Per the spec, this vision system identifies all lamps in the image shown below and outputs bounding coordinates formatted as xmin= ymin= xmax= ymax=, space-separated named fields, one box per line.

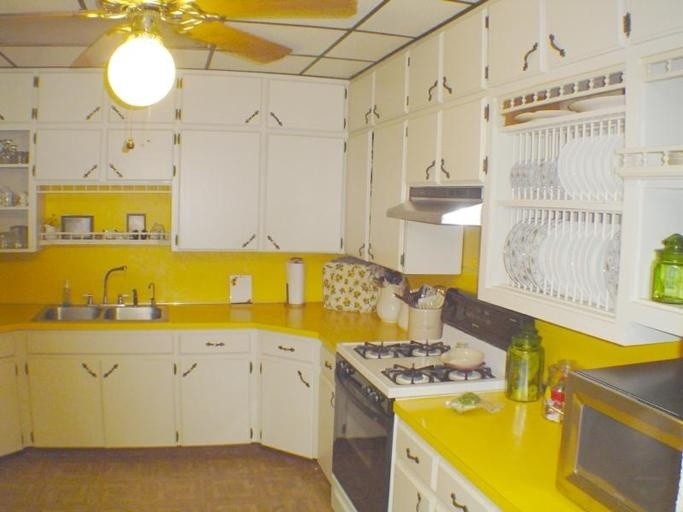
xmin=107 ymin=19 xmax=176 ymax=108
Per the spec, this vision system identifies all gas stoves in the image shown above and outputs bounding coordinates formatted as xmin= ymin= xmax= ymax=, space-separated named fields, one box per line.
xmin=336 ymin=323 xmax=506 ymax=416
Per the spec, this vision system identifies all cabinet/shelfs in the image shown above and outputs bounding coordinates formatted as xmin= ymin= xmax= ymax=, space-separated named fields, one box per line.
xmin=487 ymin=1 xmax=625 ymax=87
xmin=408 ymin=9 xmax=486 ymax=192
xmin=178 ymin=330 xmax=258 ymax=447
xmin=259 ymin=334 xmax=318 ymax=461
xmin=38 ymin=73 xmax=175 ymax=187
xmin=176 ymin=69 xmax=345 ymax=254
xmin=347 ymin=53 xmax=408 ymax=273
xmin=1 ymin=333 xmax=23 ymax=459
xmin=26 ymin=329 xmax=178 ymax=448
xmin=1 ymin=69 xmax=38 ymax=252
xmin=317 ymin=347 xmax=338 ymax=483
xmin=625 ymin=1 xmax=683 ymax=335
xmin=388 ymin=420 xmax=498 ymax=512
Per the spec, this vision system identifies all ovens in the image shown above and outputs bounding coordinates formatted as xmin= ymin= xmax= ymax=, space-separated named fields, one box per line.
xmin=332 ymin=372 xmax=395 ymax=511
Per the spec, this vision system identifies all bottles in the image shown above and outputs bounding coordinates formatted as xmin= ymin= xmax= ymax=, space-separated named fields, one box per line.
xmin=506 ymin=328 xmax=545 ymax=402
xmin=650 ymin=233 xmax=683 ymax=304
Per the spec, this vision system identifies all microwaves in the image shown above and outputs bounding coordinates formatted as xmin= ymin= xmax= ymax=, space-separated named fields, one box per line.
xmin=556 ymin=357 xmax=683 ymax=512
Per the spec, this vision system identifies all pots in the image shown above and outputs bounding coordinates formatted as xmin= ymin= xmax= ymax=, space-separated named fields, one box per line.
xmin=439 ymin=342 xmax=485 ymax=370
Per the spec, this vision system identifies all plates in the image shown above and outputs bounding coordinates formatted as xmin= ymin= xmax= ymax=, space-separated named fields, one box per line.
xmin=514 ymin=110 xmax=575 ymax=120
xmin=502 ymin=134 xmax=624 ymax=302
xmin=568 ymin=95 xmax=625 ymax=112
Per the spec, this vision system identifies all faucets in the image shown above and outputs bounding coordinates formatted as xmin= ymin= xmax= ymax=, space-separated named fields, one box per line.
xmin=102 ymin=264 xmax=127 ymax=304
xmin=148 ymin=282 xmax=156 ymax=306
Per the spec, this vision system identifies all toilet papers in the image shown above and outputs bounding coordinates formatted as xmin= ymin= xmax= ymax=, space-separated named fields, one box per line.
xmin=286 ymin=258 xmax=305 ymax=309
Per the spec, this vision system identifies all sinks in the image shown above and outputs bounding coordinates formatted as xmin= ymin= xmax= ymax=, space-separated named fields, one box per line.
xmin=104 ymin=306 xmax=162 ymax=321
xmin=35 ymin=303 xmax=102 ymax=321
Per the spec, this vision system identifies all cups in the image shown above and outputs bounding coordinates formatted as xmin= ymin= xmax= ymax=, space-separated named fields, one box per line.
xmin=408 ymin=307 xmax=443 ymax=340
xmin=376 ymin=287 xmax=402 ymax=322
xmin=397 ymin=301 xmax=409 ymax=331
xmin=542 ymin=361 xmax=581 ymax=422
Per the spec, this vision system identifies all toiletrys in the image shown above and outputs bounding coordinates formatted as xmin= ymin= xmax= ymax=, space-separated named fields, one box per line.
xmin=62 ymin=280 xmax=72 ymax=305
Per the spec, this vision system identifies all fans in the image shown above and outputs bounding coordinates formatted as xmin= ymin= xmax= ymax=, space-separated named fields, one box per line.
xmin=0 ymin=0 xmax=357 ymax=69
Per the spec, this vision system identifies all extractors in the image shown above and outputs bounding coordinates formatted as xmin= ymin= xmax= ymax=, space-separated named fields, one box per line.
xmin=386 ymin=185 xmax=483 ymax=226
